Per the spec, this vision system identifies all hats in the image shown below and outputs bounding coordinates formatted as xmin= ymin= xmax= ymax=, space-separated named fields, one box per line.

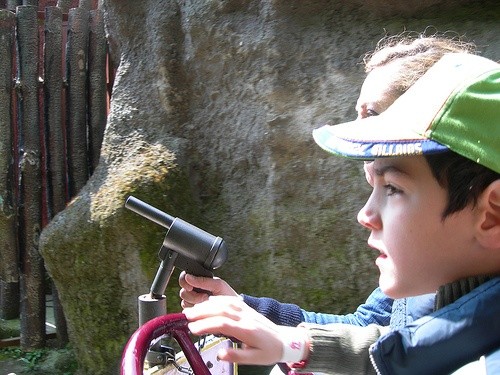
xmin=313 ymin=53 xmax=500 ymax=174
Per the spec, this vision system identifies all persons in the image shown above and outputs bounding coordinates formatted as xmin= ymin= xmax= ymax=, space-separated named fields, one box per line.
xmin=183 ymin=53 xmax=500 ymax=375
xmin=179 ymin=36 xmax=472 ymax=326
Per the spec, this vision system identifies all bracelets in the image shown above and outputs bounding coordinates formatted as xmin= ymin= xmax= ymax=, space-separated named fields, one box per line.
xmin=281 ymin=324 xmax=306 ymax=362
